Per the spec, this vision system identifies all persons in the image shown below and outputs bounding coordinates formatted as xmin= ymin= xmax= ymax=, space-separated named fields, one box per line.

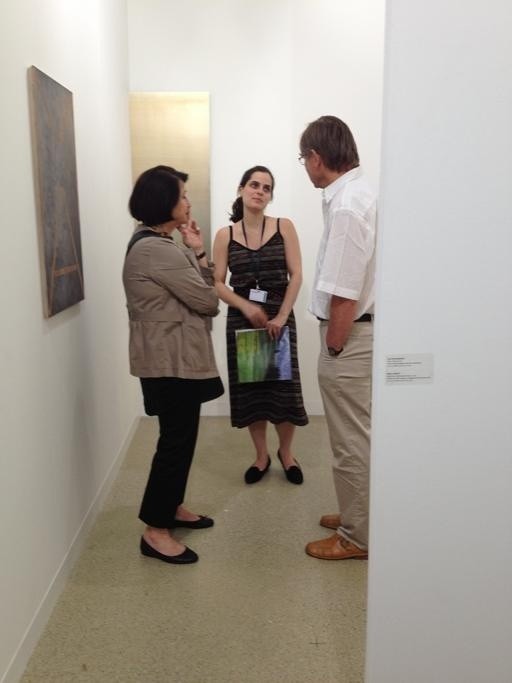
xmin=213 ymin=166 xmax=309 ymax=484
xmin=298 ymin=115 xmax=376 ymax=562
xmin=121 ymin=165 xmax=218 ymax=563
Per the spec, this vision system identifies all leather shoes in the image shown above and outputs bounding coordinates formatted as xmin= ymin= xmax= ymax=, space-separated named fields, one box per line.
xmin=245 ymin=454 xmax=271 ymax=484
xmin=305 ymin=532 xmax=368 ymax=560
xmin=140 ymin=530 xmax=199 ymax=566
xmin=277 ymin=448 xmax=303 ymax=484
xmin=319 ymin=512 xmax=343 ymax=530
xmin=172 ymin=512 xmax=214 ymax=528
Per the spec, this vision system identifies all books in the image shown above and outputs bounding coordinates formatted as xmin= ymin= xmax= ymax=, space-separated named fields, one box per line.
xmin=235 ymin=325 xmax=293 ymax=384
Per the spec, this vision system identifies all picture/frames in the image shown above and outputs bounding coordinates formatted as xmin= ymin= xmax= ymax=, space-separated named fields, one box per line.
xmin=27 ymin=64 xmax=84 ymax=320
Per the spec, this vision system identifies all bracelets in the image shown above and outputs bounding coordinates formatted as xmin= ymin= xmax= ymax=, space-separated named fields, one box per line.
xmin=197 ymin=251 xmax=206 ymax=259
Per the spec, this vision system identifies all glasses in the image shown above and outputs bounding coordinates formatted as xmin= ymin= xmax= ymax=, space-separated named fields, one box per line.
xmin=298 ymin=156 xmax=306 ymax=164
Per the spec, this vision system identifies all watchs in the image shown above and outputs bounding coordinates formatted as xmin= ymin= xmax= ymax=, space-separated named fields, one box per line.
xmin=326 ymin=347 xmax=343 ymax=356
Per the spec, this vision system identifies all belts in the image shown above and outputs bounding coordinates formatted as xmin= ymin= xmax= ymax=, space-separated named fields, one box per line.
xmin=314 ymin=314 xmax=372 ymax=323
